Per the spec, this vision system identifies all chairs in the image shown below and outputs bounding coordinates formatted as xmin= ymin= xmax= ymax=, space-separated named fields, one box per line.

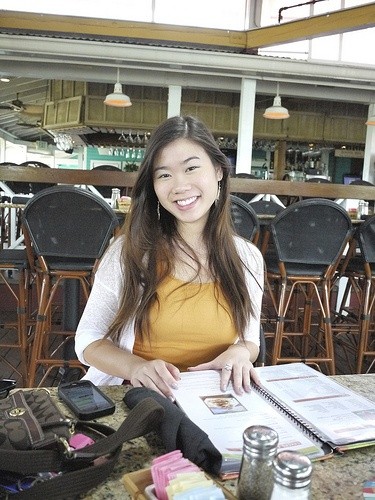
xmin=0 ymin=160 xmax=375 ymax=390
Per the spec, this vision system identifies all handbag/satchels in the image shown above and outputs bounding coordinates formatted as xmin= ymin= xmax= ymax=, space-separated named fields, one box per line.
xmin=0 ymin=388 xmax=164 ymax=500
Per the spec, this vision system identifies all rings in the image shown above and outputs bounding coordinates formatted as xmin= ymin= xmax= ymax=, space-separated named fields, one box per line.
xmin=223 ymin=364 xmax=233 ymax=371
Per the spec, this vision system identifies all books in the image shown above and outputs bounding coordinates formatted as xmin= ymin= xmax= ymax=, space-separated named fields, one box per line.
xmin=159 ymin=361 xmax=375 ymax=481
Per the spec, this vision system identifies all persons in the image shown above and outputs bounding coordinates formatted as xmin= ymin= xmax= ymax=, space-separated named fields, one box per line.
xmin=73 ymin=116 xmax=266 ymax=403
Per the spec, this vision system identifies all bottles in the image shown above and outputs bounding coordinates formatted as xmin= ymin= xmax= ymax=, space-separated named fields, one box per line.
xmin=111 ymin=188 xmax=120 ymax=209
xmin=358 ymin=202 xmax=369 ymax=220
xmin=349 ymin=208 xmax=358 ymax=220
xmin=236 ymin=426 xmax=279 ymax=500
xmin=270 ymin=450 xmax=313 ymax=500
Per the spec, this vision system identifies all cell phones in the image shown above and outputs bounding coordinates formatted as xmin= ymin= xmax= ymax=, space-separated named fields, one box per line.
xmin=59 ymin=380 xmax=117 ymax=421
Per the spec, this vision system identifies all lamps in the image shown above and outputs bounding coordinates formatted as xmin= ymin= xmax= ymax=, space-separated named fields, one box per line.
xmin=263 ymin=81 xmax=291 ymax=119
xmin=365 ymin=106 xmax=375 ymax=126
xmin=11 ymin=94 xmax=22 ymax=109
xmin=103 ymin=68 xmax=133 ymax=108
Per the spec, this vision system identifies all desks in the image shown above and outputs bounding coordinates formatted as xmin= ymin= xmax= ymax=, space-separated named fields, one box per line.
xmin=9 ymin=373 xmax=375 ymax=500
